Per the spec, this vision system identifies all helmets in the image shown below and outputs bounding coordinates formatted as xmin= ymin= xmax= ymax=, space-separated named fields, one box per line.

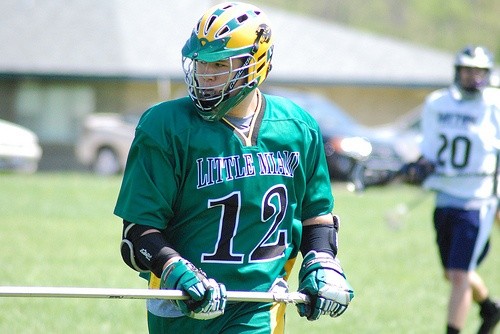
xmin=181 ymin=2 xmax=274 ymax=121
xmin=453 ymin=46 xmax=493 ymax=101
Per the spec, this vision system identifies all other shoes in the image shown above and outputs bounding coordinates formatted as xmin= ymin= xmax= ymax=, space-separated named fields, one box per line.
xmin=478 ymin=303 xmax=500 ymax=334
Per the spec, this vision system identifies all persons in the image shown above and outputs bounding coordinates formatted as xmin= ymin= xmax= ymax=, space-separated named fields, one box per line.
xmin=113 ymin=1 xmax=355 ymax=334
xmin=402 ymin=42 xmax=500 ymax=334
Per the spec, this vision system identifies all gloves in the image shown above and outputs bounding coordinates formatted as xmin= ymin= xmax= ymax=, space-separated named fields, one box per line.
xmin=159 ymin=259 xmax=228 ymax=320
xmin=295 ymin=250 xmax=355 ymax=321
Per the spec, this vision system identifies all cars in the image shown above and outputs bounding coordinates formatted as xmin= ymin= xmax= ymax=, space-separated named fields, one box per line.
xmin=260 ymin=87 xmax=397 ymax=181
xmin=74 ymin=111 xmax=147 ymax=176
xmin=379 ymin=106 xmax=432 ymax=185
xmin=1 ymin=120 xmax=43 ymax=173
